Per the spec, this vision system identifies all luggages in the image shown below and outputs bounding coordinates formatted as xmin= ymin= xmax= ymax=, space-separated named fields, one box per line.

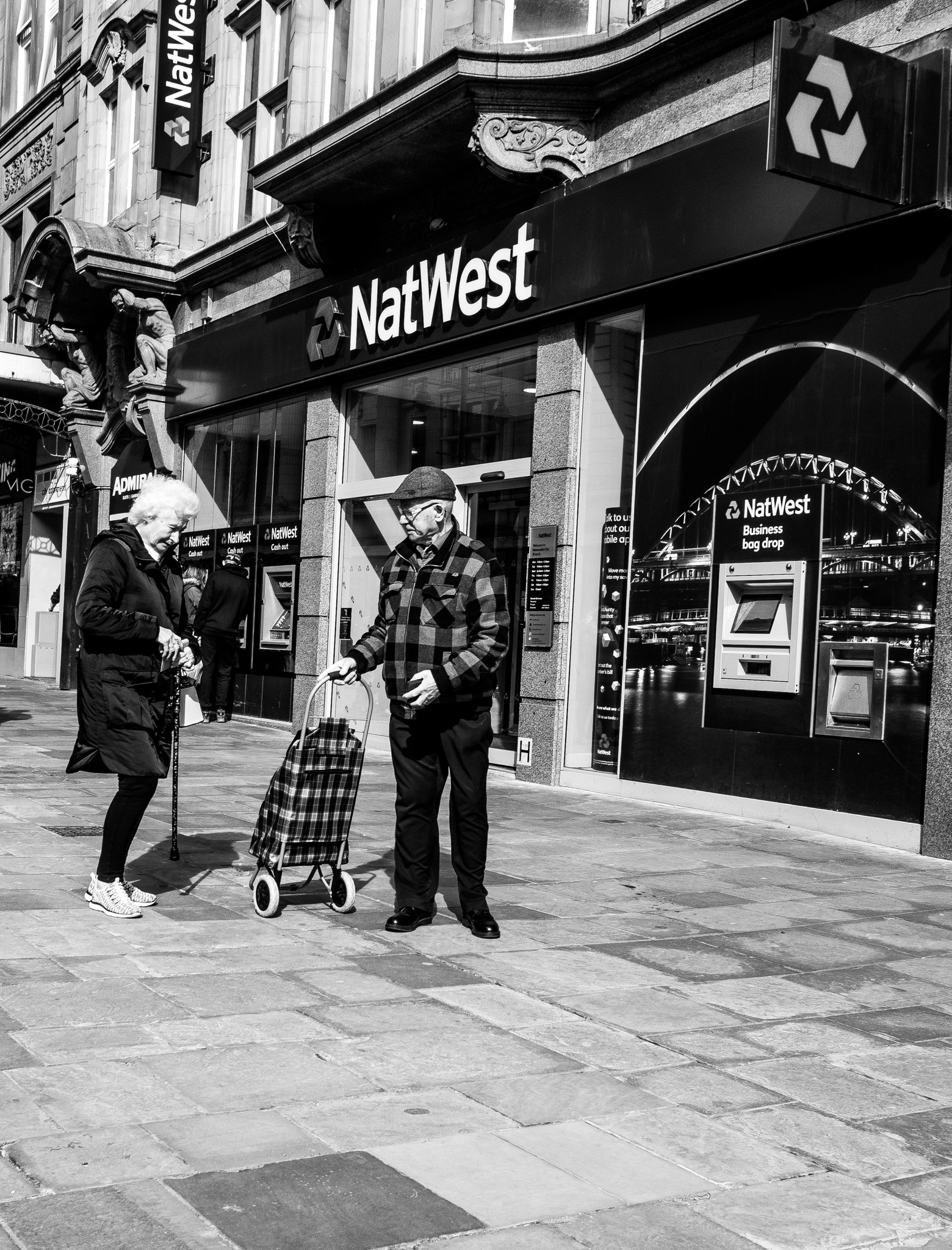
xmin=247 ymin=671 xmax=374 ymax=918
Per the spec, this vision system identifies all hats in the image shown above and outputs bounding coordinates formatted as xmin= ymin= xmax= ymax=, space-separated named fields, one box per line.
xmin=224 ymin=554 xmax=241 ymax=566
xmin=389 ymin=467 xmax=457 ymax=501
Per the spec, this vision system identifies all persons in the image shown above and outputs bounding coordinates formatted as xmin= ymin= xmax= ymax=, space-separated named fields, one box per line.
xmin=65 ymin=476 xmax=204 ymax=918
xmin=317 ymin=468 xmax=509 ymax=938
xmin=37 ymin=323 xmax=107 ymax=412
xmin=182 ymin=566 xmax=207 ymax=624
xmin=111 ymin=289 xmax=176 ymax=384
xmin=193 ymin=553 xmax=252 ymax=723
xmin=49 ymin=584 xmax=60 ymax=611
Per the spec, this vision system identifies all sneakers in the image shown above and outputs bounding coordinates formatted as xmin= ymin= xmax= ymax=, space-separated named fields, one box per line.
xmin=84 ymin=872 xmax=157 ymax=907
xmin=88 ymin=875 xmax=142 ymax=918
xmin=217 ymin=710 xmax=227 ymax=722
xmin=201 ymin=710 xmax=210 ymax=723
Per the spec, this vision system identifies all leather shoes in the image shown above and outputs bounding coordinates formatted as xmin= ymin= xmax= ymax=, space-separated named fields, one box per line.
xmin=384 ymin=905 xmax=434 ymax=930
xmin=463 ymin=907 xmax=500 ymax=938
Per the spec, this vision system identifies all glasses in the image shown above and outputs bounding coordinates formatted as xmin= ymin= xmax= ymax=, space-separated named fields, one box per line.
xmin=397 ymin=503 xmax=447 ymax=518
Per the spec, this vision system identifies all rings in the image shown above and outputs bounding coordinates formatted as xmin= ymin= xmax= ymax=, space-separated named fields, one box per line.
xmin=173 ymin=641 xmax=178 ymax=644
xmin=185 ymin=656 xmax=189 ymax=662
